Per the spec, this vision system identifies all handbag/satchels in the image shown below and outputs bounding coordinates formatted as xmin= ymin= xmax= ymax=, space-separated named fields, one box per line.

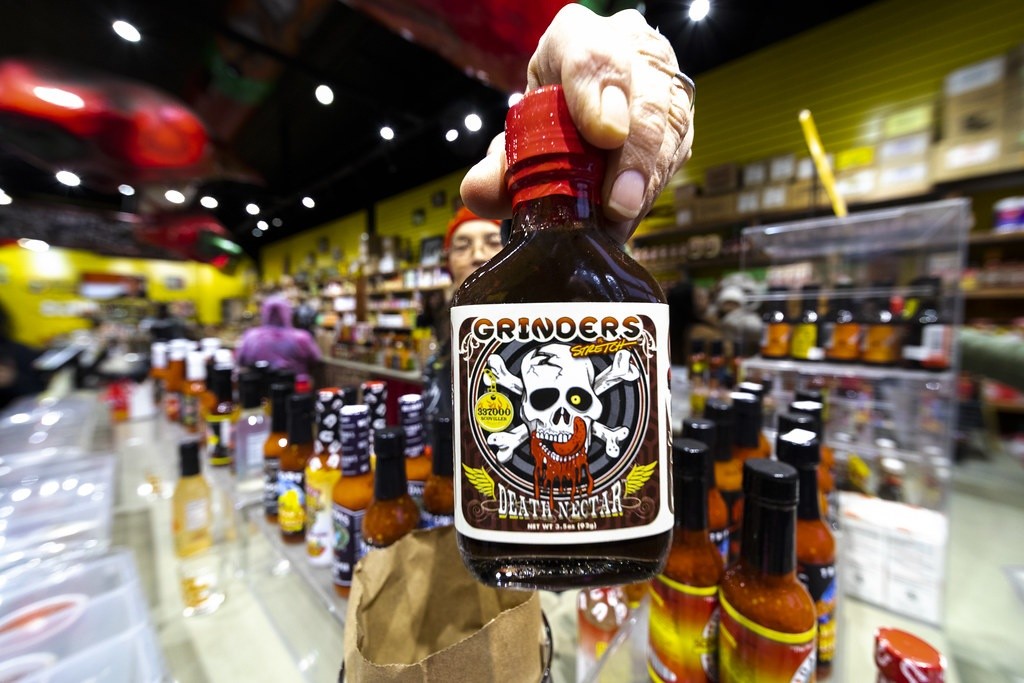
xmin=336 ymin=524 xmax=554 ymax=683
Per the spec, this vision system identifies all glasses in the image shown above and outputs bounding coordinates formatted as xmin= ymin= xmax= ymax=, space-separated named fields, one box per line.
xmin=443 ymin=233 xmax=502 ymax=254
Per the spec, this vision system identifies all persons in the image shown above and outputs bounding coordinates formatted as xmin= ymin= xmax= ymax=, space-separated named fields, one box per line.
xmin=413 ymin=0 xmax=697 ymax=287
xmin=236 ymin=296 xmax=323 ymax=378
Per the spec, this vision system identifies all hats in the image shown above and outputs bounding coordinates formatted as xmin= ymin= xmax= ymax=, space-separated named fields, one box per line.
xmin=444 ymin=206 xmax=503 ymax=251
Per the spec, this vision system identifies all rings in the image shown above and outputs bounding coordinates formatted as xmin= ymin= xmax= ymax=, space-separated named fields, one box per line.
xmin=675 ymin=71 xmax=695 ymax=111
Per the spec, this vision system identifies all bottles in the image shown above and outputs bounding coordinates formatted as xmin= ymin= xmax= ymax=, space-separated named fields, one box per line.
xmin=776 ymin=429 xmax=836 ymax=681
xmin=689 ymin=351 xmax=707 ymax=414
xmin=709 ymin=355 xmax=727 ymax=399
xmin=794 ymin=388 xmax=835 ymax=472
xmin=576 ymin=586 xmax=633 ymax=683
xmin=778 ymin=414 xmax=830 ymax=521
xmin=737 ymin=381 xmax=772 ymax=459
xmin=742 ymin=273 xmax=954 ymax=370
xmin=705 ymin=402 xmax=743 ymax=536
xmin=875 ymin=628 xmax=944 ymax=683
xmin=730 ymin=393 xmax=766 ymax=465
xmin=149 ymin=336 xmax=452 ymax=618
xmin=720 ymin=459 xmax=817 ymax=683
xmin=645 ymin=438 xmax=726 ymax=683
xmin=730 ymin=357 xmax=746 ymax=392
xmin=790 ymin=401 xmax=832 ymax=495
xmin=836 ymin=429 xmax=952 ymax=512
xmin=682 ymin=418 xmax=728 ymax=569
xmin=451 ymin=86 xmax=676 ymax=590
